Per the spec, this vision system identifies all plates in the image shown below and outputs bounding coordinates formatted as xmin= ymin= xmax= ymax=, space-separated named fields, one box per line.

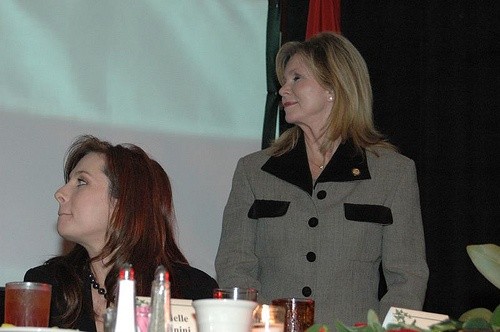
xmin=0 ymin=324 xmax=86 ymax=332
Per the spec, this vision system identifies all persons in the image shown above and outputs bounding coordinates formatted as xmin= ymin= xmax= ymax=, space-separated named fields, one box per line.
xmin=23 ymin=135 xmax=220 ymax=332
xmin=214 ymin=30 xmax=429 ymax=332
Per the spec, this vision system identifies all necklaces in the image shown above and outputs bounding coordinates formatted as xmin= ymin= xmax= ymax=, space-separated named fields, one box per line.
xmin=318 ymin=161 xmax=325 ymax=171
xmin=88 ymin=271 xmax=108 ymax=301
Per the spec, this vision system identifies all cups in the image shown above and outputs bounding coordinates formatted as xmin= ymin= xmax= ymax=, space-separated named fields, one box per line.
xmin=273 ymin=298 xmax=315 ymax=332
xmin=211 ymin=288 xmax=258 ymax=303
xmin=191 ymin=298 xmax=259 ymax=332
xmin=248 ymin=302 xmax=286 ymax=332
xmin=4 ymin=282 xmax=52 ymax=328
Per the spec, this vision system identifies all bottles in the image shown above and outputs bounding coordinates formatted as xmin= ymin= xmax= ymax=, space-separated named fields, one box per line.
xmin=109 ymin=262 xmax=138 ymax=332
xmin=149 ymin=264 xmax=172 ymax=332
